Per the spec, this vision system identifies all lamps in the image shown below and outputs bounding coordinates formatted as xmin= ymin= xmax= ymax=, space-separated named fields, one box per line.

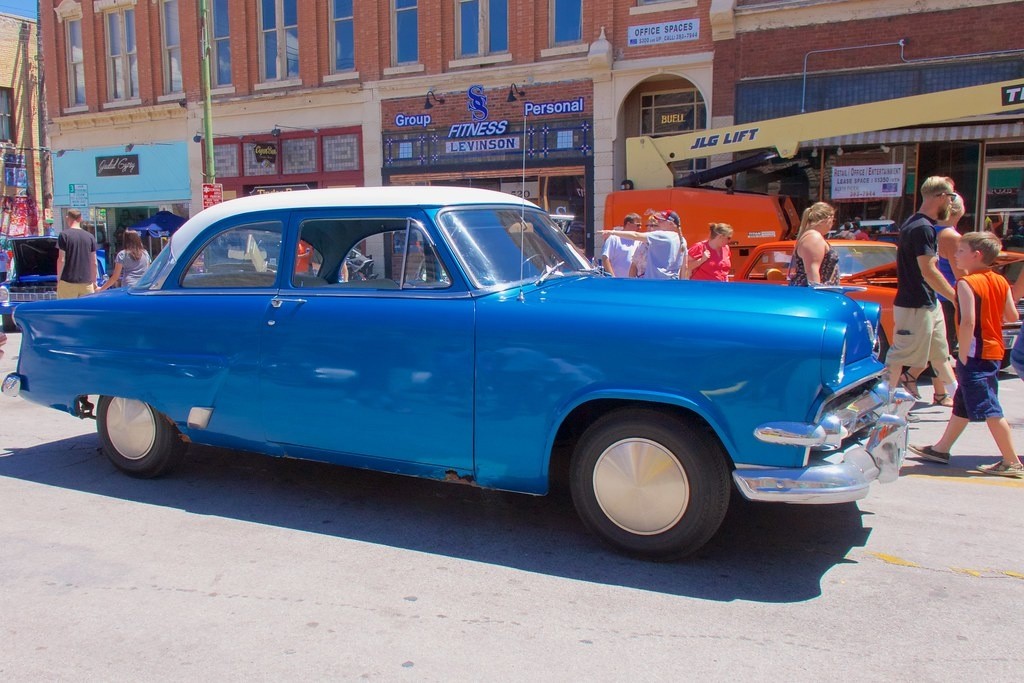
xmin=507 ymin=83 xmax=525 ymax=103
xmin=270 ymin=124 xmax=319 ymax=137
xmin=125 ymin=143 xmax=171 ymax=153
xmin=811 ymin=147 xmax=818 ymax=157
xmin=193 ymin=132 xmax=243 ymax=143
xmin=55 ymin=150 xmax=83 ymax=158
xmin=880 ymin=144 xmax=890 ymax=153
xmin=837 ymin=146 xmax=844 ymax=156
xmin=424 ymin=91 xmax=445 ymax=109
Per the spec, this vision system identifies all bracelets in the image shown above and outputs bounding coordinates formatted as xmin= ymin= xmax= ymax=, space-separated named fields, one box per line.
xmin=696 ymin=258 xmax=703 ymax=265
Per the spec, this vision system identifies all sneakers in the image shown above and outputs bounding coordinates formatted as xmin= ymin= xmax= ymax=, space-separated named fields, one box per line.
xmin=908 ymin=443 xmax=949 ymax=463
xmin=976 ymin=460 xmax=1024 ymax=477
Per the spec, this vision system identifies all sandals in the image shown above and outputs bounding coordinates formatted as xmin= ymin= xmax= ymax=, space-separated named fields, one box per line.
xmin=898 ymin=371 xmax=921 ymax=399
xmin=933 ymin=392 xmax=955 ymax=408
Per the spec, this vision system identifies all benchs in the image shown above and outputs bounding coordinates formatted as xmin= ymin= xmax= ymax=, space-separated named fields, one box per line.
xmin=180 ymin=272 xmax=329 ymax=291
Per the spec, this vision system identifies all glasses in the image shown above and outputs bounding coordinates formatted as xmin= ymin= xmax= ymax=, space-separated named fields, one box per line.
xmin=935 ymin=193 xmax=957 ymax=202
xmin=630 ymin=222 xmax=643 ymax=227
xmin=723 ymin=235 xmax=731 ymax=240
xmin=645 ymin=223 xmax=659 ymax=229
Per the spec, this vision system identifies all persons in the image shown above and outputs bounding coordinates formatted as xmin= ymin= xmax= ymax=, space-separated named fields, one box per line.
xmin=907 ymin=231 xmax=1024 ymax=477
xmin=984 ymin=210 xmax=996 ymax=235
xmin=687 ymin=222 xmax=734 ymax=282
xmin=855 ymin=225 xmax=870 ymax=240
xmin=295 ymin=241 xmax=313 ymax=274
xmin=852 ymin=222 xmax=861 ymax=235
xmin=834 ymin=222 xmax=856 ymax=239
xmin=0 ymin=243 xmax=11 ymax=284
xmin=788 ymin=202 xmax=840 ymax=286
xmin=1010 ymin=266 xmax=1024 ymax=381
xmin=601 ymin=212 xmax=643 ymax=278
xmin=899 ymin=192 xmax=965 ymax=405
xmin=884 ymin=176 xmax=959 ymax=398
xmin=54 ymin=208 xmax=98 ymax=299
xmin=1000 ymin=217 xmax=1024 ymax=250
xmin=95 ymin=227 xmax=151 ymax=294
xmin=595 ymin=208 xmax=688 ymax=279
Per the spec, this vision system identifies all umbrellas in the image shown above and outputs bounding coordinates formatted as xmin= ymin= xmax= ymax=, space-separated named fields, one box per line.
xmin=125 ymin=210 xmax=188 ymax=252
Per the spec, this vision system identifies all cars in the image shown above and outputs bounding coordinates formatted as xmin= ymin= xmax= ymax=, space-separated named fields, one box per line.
xmin=0 ymin=234 xmax=116 ymax=333
xmin=0 ymin=184 xmax=921 ymax=564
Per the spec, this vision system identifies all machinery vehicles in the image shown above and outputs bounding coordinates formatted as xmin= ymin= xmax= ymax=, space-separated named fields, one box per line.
xmin=597 ymin=76 xmax=1023 ymax=377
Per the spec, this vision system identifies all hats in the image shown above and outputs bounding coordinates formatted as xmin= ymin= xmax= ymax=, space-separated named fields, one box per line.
xmin=654 ymin=209 xmax=679 ymax=227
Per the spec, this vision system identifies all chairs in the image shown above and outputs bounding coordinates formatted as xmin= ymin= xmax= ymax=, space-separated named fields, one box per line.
xmin=319 ymin=278 xmax=402 ymax=290
